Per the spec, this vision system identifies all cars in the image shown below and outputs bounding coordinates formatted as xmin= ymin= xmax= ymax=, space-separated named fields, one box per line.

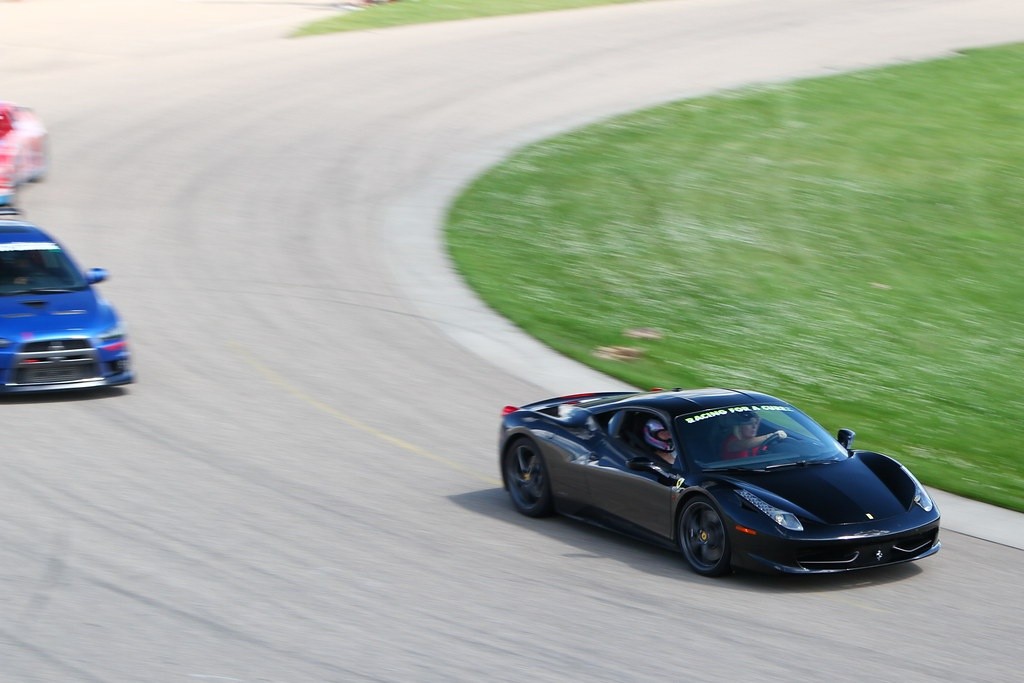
xmin=1 ymin=220 xmax=137 ymax=395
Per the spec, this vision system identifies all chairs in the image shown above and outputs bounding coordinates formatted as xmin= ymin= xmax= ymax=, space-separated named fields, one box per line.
xmin=708 ymin=416 xmax=737 ymax=462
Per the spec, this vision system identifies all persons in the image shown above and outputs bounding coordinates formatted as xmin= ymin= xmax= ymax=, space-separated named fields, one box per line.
xmin=644 ymin=419 xmax=678 ymax=470
xmin=724 ymin=411 xmax=786 ymax=458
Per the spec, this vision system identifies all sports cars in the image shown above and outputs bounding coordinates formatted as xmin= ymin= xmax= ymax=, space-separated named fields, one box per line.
xmin=498 ymin=387 xmax=942 ymax=578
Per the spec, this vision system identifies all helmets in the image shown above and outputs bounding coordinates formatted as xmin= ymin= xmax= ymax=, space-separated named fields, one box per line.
xmin=642 ymin=417 xmax=676 ymax=453
